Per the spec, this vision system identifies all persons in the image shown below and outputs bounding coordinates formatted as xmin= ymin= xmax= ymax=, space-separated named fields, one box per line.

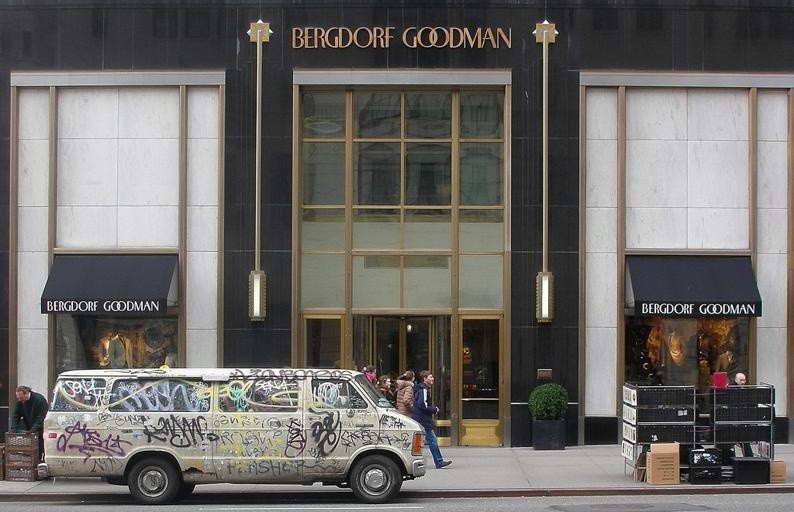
xmin=410 ymin=370 xmax=452 ymax=469
xmin=373 ymin=374 xmax=394 ymax=405
xmin=7 ymin=384 xmax=49 ymax=461
xmin=56 ymin=326 xmax=176 ymax=367
xmin=361 ymin=365 xmax=377 ymax=386
xmin=396 ymin=370 xmax=415 ymax=416
xmin=664 ymin=316 xmax=748 ymax=373
xmin=728 ymin=372 xmax=754 ymax=457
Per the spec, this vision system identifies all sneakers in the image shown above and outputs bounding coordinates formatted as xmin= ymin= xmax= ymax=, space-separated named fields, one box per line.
xmin=436 ymin=460 xmax=453 ymax=469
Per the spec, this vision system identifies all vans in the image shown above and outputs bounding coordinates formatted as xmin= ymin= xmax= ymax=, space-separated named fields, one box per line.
xmin=37 ymin=367 xmax=427 ymax=504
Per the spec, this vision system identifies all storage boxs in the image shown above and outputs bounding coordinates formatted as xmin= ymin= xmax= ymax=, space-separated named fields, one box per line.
xmin=645 ymin=442 xmax=787 ymax=484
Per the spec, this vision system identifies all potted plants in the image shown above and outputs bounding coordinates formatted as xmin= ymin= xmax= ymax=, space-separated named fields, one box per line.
xmin=529 ymin=383 xmax=569 ymax=449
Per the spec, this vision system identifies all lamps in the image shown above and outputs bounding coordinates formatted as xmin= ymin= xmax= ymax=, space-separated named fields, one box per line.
xmin=248 ymin=270 xmax=267 ymax=322
xmin=534 ymin=272 xmax=554 ymax=323
